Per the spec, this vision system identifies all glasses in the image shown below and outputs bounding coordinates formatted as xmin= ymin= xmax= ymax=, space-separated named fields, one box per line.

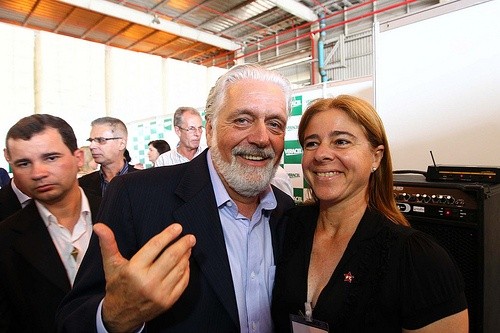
xmin=177 ymin=126 xmax=205 ymax=133
xmin=86 ymin=137 xmax=124 ymax=144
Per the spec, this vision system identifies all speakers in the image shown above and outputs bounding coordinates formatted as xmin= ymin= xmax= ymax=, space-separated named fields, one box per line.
xmin=392 ymin=175 xmax=500 ymax=333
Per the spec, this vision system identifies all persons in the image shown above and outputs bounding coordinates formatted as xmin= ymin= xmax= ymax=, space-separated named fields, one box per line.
xmin=156 ymin=107 xmax=206 ymax=167
xmin=0 ymin=178 xmax=32 ymax=221
xmin=271 ymin=95 xmax=469 ymax=333
xmin=77 ymin=146 xmax=97 ymax=178
xmin=0 ymin=114 xmax=103 ymax=333
xmin=49 ymin=62 xmax=297 ymax=332
xmin=271 ymin=166 xmax=294 ymax=198
xmin=148 ymin=140 xmax=170 ymax=167
xmin=77 ymin=116 xmax=141 ymax=199
